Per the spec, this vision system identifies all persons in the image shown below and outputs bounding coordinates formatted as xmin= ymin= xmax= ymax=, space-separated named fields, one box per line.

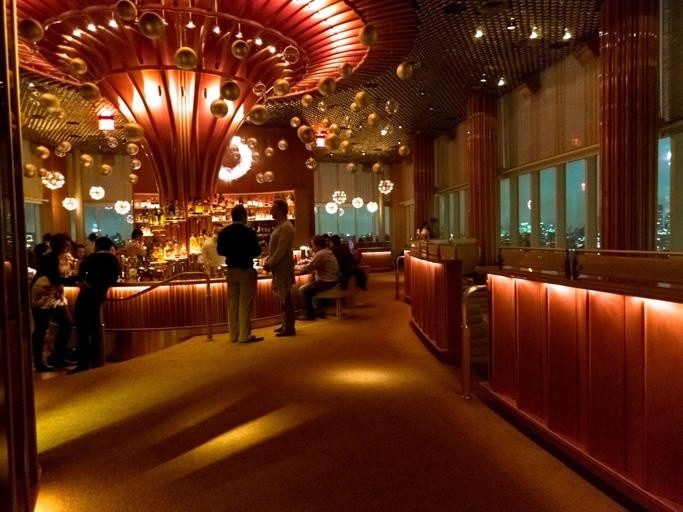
xmin=215 ymin=204 xmax=265 ymax=344
xmin=292 ymin=232 xmax=361 ymax=320
xmin=27 ymin=228 xmax=175 ymax=282
xmin=199 ymin=221 xmax=225 ymax=277
xmin=29 ymin=232 xmax=75 ymax=374
xmin=421 ymin=219 xmax=435 ymax=240
xmin=66 ymin=236 xmax=121 ymax=374
xmin=261 ymin=199 xmax=295 ymax=337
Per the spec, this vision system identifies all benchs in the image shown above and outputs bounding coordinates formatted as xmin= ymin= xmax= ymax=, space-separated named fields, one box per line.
xmin=314 ymin=267 xmax=370 ymax=319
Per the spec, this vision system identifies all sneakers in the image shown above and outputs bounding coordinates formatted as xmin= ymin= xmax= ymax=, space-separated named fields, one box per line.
xmin=273 ymin=323 xmax=297 ymax=337
xmin=229 ymin=334 xmax=265 ymax=344
